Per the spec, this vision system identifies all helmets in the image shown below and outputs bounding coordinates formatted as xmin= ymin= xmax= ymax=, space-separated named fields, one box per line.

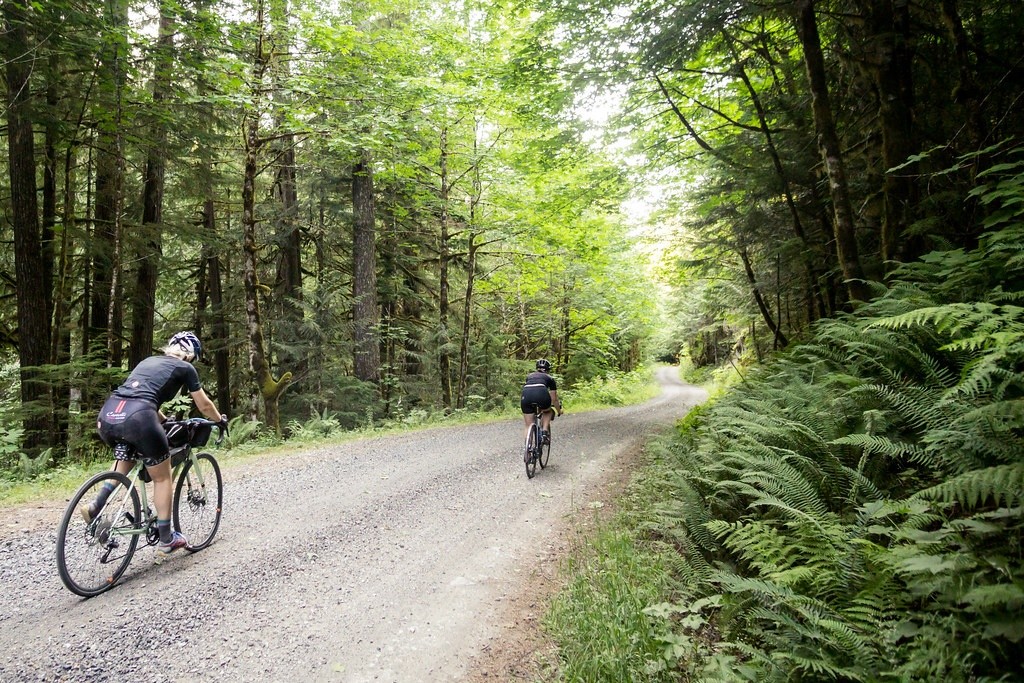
xmin=535 ymin=360 xmax=550 ymax=370
xmin=167 ymin=330 xmax=203 ymax=364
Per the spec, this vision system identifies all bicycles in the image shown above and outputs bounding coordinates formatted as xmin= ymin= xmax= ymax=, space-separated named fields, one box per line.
xmin=518 ymin=397 xmax=564 ymax=482
xmin=55 ymin=415 xmax=224 ymax=596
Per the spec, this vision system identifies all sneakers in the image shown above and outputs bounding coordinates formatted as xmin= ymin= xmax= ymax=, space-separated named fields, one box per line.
xmin=157 ymin=532 xmax=188 ymax=558
xmin=81 ymin=501 xmax=99 ymax=526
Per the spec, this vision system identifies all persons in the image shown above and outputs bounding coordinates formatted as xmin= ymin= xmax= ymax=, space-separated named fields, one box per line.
xmin=79 ymin=330 xmax=228 ymax=556
xmin=520 ymin=359 xmax=563 ymax=463
